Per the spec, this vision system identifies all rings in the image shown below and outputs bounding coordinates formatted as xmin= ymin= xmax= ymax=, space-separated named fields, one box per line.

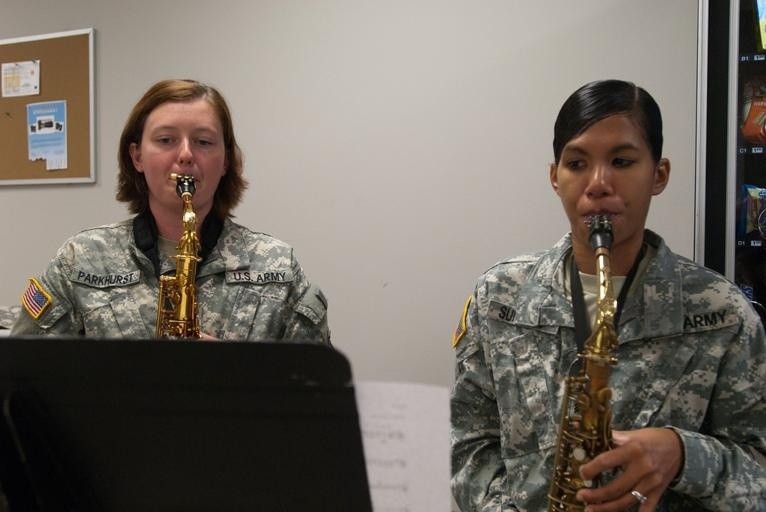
xmin=630 ymin=490 xmax=648 ymax=504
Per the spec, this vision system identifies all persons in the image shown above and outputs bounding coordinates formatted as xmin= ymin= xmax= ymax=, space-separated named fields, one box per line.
xmin=451 ymin=78 xmax=766 ymax=511
xmin=8 ymin=78 xmax=331 ymax=348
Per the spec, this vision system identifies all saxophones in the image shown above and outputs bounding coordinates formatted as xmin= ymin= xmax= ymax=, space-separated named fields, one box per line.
xmin=546 ymin=213 xmax=618 ymax=512
xmin=156 ymin=173 xmax=201 ymax=341
